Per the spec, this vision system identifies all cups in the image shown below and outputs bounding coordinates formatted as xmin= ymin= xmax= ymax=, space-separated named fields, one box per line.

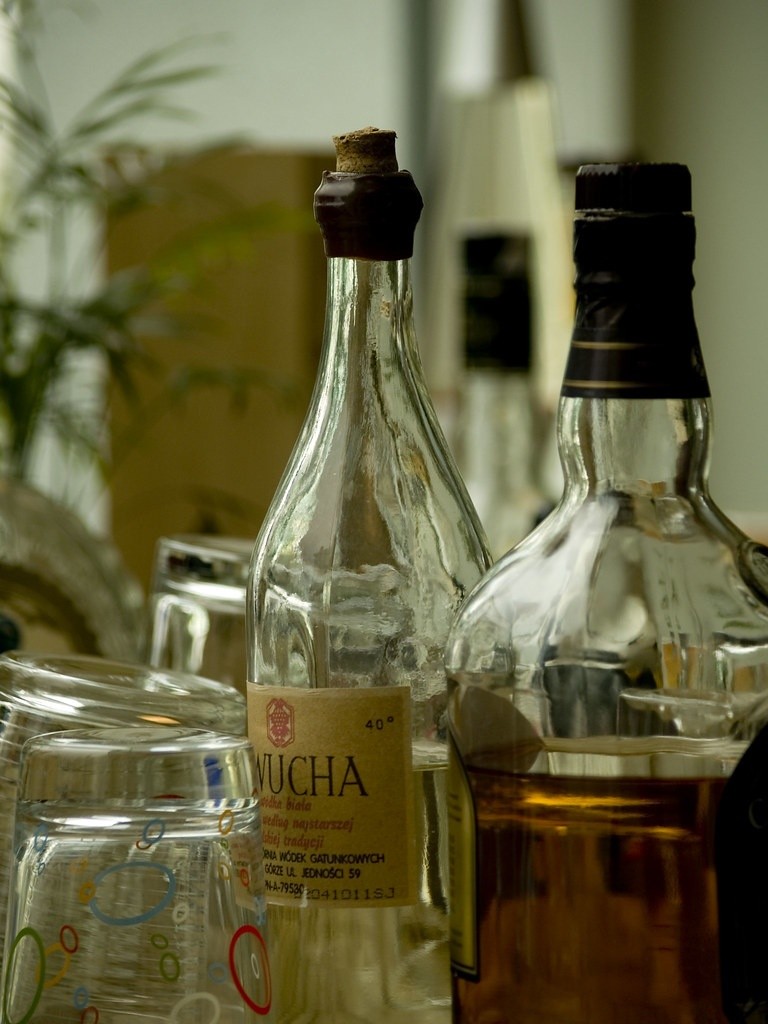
xmin=147 ymin=535 xmax=254 ymax=696
xmin=0 ymin=729 xmax=272 ymax=1024
xmin=0 ymin=650 xmax=246 ymax=976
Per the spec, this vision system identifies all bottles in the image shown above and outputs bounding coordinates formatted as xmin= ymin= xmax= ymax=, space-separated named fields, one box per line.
xmin=446 ymin=162 xmax=767 ymax=1024
xmin=246 ymin=130 xmax=490 ymax=1024
xmin=452 ymin=222 xmax=548 ymax=564
